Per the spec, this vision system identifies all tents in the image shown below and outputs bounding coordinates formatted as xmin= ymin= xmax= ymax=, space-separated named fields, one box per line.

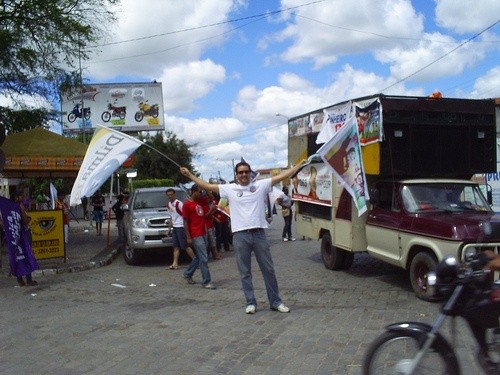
xmin=0 ymin=127 xmax=136 ymax=247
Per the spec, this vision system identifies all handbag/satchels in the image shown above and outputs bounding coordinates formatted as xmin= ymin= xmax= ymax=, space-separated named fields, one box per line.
xmin=281 ymin=208 xmax=290 ymax=217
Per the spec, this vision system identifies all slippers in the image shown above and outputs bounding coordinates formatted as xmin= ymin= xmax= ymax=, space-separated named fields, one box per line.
xmin=167 ymin=265 xmax=178 ymax=270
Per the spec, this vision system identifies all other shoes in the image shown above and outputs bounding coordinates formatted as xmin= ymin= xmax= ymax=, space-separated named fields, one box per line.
xmin=217 ymin=250 xmax=224 ymax=252
xmin=212 ymin=255 xmax=220 ymax=259
xmin=185 ymin=277 xmax=196 ymax=284
xmin=289 ymin=237 xmax=296 ymax=240
xmin=203 ymin=282 xmax=216 ymax=289
xmin=28 ymin=281 xmax=38 ymax=286
xmin=246 ymin=304 xmax=255 ymax=313
xmin=283 ymin=237 xmax=288 ymax=242
xmin=270 ymin=303 xmax=290 ymax=313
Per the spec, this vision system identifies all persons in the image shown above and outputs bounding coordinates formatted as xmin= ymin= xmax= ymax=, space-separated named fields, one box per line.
xmin=111 ymin=194 xmax=125 ymax=242
xmin=167 ymin=188 xmax=233 ymax=269
xmin=293 ymin=104 xmax=381 ymax=200
xmin=182 ymin=183 xmax=217 ymax=289
xmin=276 ymin=186 xmax=297 ymax=242
xmin=180 ymin=159 xmax=308 ymax=314
xmin=55 ymin=197 xmax=70 ymax=243
xmin=14 ymin=191 xmax=37 ymax=287
xmin=82 ymin=195 xmax=88 ymax=219
xmin=90 ymin=189 xmax=105 ymax=236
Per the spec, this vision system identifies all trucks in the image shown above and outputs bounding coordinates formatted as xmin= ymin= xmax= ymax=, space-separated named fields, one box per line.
xmin=286 ymin=92 xmax=500 ymax=302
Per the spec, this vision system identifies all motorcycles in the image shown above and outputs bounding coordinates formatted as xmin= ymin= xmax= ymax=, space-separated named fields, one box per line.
xmin=101 ymin=98 xmax=127 ymax=122
xmin=135 ymin=99 xmax=159 ymax=122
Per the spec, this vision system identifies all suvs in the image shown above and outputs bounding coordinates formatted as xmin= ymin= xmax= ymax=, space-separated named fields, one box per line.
xmin=119 ymin=186 xmax=197 ymax=265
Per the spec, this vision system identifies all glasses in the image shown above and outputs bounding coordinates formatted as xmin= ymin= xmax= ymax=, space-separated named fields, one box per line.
xmin=239 ymin=171 xmax=249 ymax=174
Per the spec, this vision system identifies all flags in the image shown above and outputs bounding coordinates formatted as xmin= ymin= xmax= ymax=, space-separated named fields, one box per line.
xmin=0 ymin=189 xmax=38 ymax=277
xmin=316 ymin=115 xmax=370 ymax=217
xmin=70 ymin=125 xmax=141 ymax=206
xmin=217 ymin=156 xmax=287 ymax=224
xmin=50 ymin=182 xmax=58 ymax=210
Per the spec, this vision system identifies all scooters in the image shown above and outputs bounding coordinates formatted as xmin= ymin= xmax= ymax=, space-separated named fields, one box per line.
xmin=67 ymin=100 xmax=92 ymax=123
xmin=360 ymin=241 xmax=500 ymax=375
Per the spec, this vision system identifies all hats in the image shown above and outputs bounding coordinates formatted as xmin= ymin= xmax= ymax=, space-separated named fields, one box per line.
xmin=10 ymin=186 xmax=24 ymax=195
xmin=117 ymin=195 xmax=125 ymax=199
